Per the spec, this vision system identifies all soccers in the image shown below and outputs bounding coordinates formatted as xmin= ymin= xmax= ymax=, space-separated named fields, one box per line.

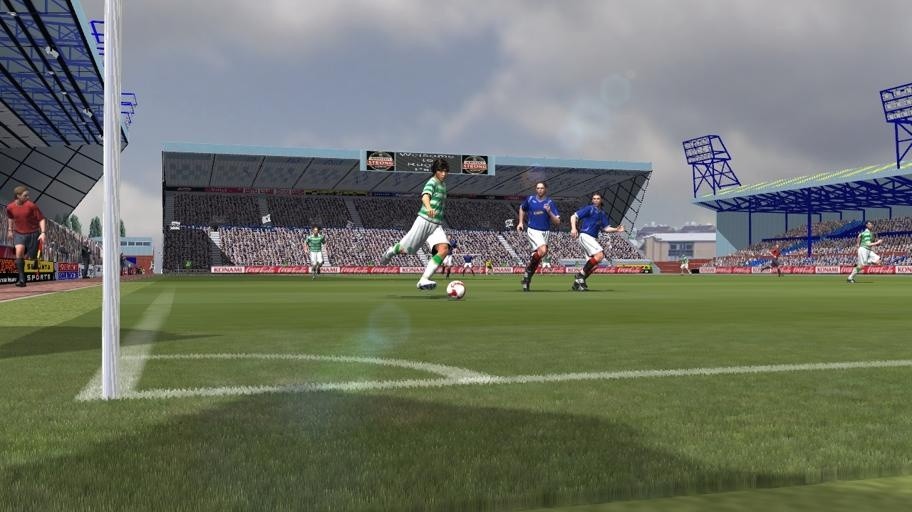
xmin=445 ymin=281 xmax=466 ymax=299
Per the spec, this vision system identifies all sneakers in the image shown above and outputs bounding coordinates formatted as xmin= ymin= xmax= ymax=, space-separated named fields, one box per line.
xmin=571 ymin=282 xmax=580 ymax=291
xmin=523 ymin=283 xmax=529 ymax=292
xmin=524 ymin=267 xmax=532 ymax=283
xmin=416 ymin=278 xmax=437 ymax=290
xmin=380 ymin=247 xmax=394 ymax=266
xmin=15 ymin=280 xmax=26 ymax=286
xmin=575 ymin=272 xmax=587 ymax=290
xmin=847 ymin=277 xmax=856 ymax=283
